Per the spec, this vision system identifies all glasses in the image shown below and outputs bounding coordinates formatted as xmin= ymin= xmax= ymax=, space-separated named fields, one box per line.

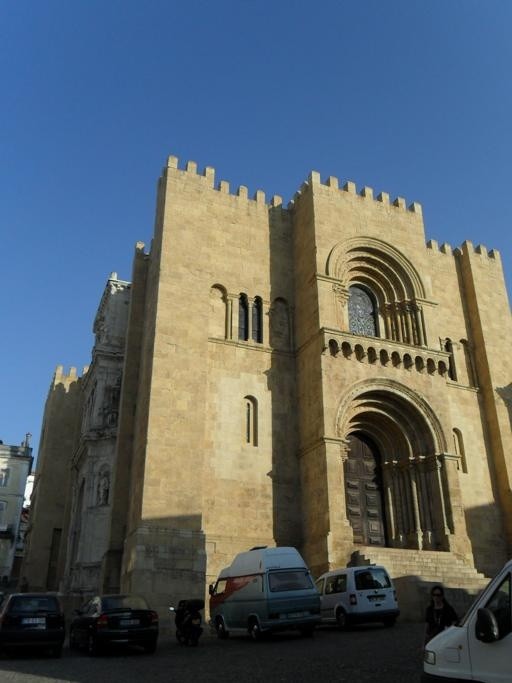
xmin=432 ymin=593 xmax=440 ymax=596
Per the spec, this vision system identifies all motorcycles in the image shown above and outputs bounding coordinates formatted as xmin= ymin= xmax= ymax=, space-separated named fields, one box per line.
xmin=169 ymin=599 xmax=205 ymax=647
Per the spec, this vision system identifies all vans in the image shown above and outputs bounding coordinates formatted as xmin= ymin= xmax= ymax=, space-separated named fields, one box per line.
xmin=422 ymin=560 xmax=511 ymax=677
xmin=208 ymin=545 xmax=321 ymax=645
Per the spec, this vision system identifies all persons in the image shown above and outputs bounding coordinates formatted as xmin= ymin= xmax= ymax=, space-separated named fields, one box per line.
xmin=422 ymin=585 xmax=457 ymax=662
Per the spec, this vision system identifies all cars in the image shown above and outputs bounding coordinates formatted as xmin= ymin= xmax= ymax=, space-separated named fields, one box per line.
xmin=314 ymin=565 xmax=400 ymax=633
xmin=69 ymin=593 xmax=159 ymax=658
xmin=0 ymin=592 xmax=66 ymax=657
